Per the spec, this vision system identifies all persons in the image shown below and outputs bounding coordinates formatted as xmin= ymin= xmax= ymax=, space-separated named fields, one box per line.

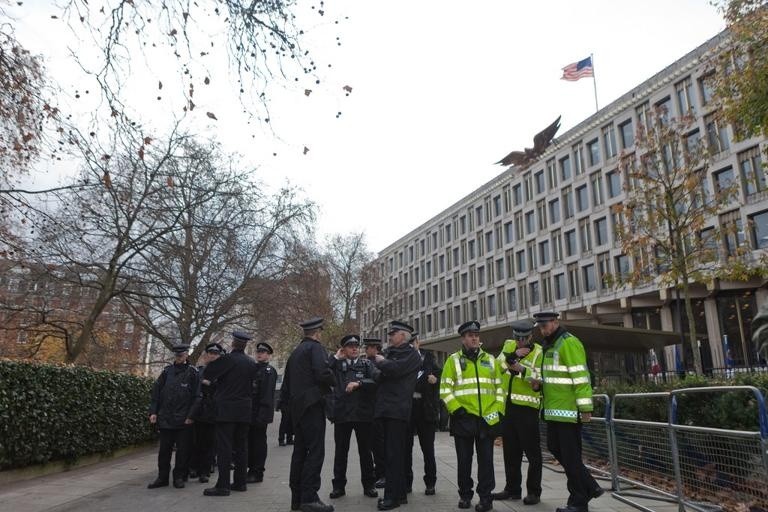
xmin=327 ymin=385 xmax=337 ymax=424
xmin=372 ymin=320 xmax=420 ymax=510
xmin=283 ymin=318 xmax=336 ymax=512
xmin=406 ymin=332 xmax=457 ymax=498
xmin=492 ymin=320 xmax=545 ymax=505
xmin=533 ymin=312 xmax=605 ymax=512
xmin=328 ymin=334 xmax=380 ymax=499
xmin=361 ymin=337 xmax=389 ymax=488
xmin=439 ymin=321 xmax=506 ymax=512
xmin=275 ymin=385 xmax=294 ymax=446
xmin=148 ymin=331 xmax=277 ymax=496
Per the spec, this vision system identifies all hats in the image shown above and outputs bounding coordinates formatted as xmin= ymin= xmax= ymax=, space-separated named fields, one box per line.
xmin=299 ymin=318 xmax=325 ymax=330
xmin=171 ymin=330 xmax=274 ymax=356
xmin=340 ymin=320 xmax=420 ymax=348
xmin=510 ymin=319 xmax=534 ymax=338
xmin=533 ymin=313 xmax=559 ymax=327
xmin=458 ymin=321 xmax=480 ymax=336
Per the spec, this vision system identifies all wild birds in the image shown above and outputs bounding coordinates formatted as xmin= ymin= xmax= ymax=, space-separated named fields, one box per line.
xmin=495 ymin=115 xmax=561 ymax=166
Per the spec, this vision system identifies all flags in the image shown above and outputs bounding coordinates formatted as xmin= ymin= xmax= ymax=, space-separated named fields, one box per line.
xmin=725 ymin=336 xmax=735 ymax=369
xmin=648 ymin=347 xmax=662 ymax=375
xmin=675 ymin=344 xmax=684 ymax=374
xmin=560 ymin=56 xmax=594 ymax=81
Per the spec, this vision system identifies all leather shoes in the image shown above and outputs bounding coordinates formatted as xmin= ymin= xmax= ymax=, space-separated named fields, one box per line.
xmin=458 ymin=494 xmax=473 ymax=508
xmin=291 ymin=499 xmax=334 ymax=512
xmin=524 ymin=494 xmax=540 ymax=504
xmin=280 ymin=440 xmax=294 ymax=446
xmin=556 ymin=505 xmax=589 ymax=512
xmin=475 ymin=500 xmax=492 ymax=511
xmin=492 ymin=490 xmax=521 ymax=500
xmin=147 ymin=461 xmax=263 ymax=496
xmin=425 ymin=487 xmax=435 ymax=494
xmin=364 ymin=478 xmax=412 ymax=510
xmin=330 ymin=489 xmax=345 ymax=498
xmin=588 ymin=487 xmax=603 ymax=502
